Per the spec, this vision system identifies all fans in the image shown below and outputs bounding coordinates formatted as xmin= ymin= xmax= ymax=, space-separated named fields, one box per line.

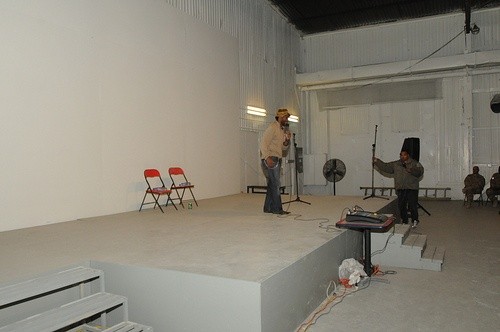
xmin=323 ymin=159 xmax=346 ymax=196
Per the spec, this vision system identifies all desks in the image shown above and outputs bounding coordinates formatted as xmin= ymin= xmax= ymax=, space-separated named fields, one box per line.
xmin=336 ymin=214 xmax=394 ymax=276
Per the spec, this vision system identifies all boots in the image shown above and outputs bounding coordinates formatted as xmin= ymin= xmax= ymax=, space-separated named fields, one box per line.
xmin=462 ymin=187 xmax=468 ymax=193
xmin=466 ymin=200 xmax=471 ymax=208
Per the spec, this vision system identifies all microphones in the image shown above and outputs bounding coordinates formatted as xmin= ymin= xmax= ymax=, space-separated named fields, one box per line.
xmin=286 ymin=124 xmax=289 ymax=131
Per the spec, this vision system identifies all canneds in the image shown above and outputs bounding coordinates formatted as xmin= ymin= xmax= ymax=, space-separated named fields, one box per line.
xmin=188 ymin=202 xmax=192 ymax=209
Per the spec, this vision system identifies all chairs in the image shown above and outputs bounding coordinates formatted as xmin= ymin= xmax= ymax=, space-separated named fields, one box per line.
xmin=486 ymin=192 xmax=500 ymax=207
xmin=138 ymin=168 xmax=178 ymax=213
xmin=462 ymin=187 xmax=483 ymax=207
xmin=166 ymin=167 xmax=198 ymax=208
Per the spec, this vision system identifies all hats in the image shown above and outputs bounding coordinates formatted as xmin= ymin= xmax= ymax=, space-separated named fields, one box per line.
xmin=275 ymin=108 xmax=289 ymax=118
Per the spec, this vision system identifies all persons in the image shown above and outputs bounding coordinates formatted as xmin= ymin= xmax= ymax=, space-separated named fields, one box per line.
xmin=373 ymin=149 xmax=424 ymax=228
xmin=462 ymin=166 xmax=485 ymax=209
xmin=486 ymin=166 xmax=500 ymax=208
xmin=261 ymin=108 xmax=291 ymax=215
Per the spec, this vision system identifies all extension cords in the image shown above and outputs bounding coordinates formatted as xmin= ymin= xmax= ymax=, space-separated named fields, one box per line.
xmin=353 ymin=285 xmax=359 ymax=291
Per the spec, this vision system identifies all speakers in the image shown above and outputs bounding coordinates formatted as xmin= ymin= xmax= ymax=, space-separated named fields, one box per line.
xmin=402 ymin=138 xmax=420 ymax=161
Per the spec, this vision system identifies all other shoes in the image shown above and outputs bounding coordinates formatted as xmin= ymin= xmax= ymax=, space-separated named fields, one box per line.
xmin=402 ymin=219 xmax=408 ymax=224
xmin=264 ymin=211 xmax=274 ymax=214
xmin=273 ymin=210 xmax=291 ymax=216
xmin=493 ymin=199 xmax=497 ymax=207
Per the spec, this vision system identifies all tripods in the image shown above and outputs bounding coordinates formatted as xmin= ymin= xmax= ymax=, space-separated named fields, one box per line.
xmin=362 ymin=125 xmax=389 ymax=200
xmin=281 ymin=133 xmax=311 ymax=205
